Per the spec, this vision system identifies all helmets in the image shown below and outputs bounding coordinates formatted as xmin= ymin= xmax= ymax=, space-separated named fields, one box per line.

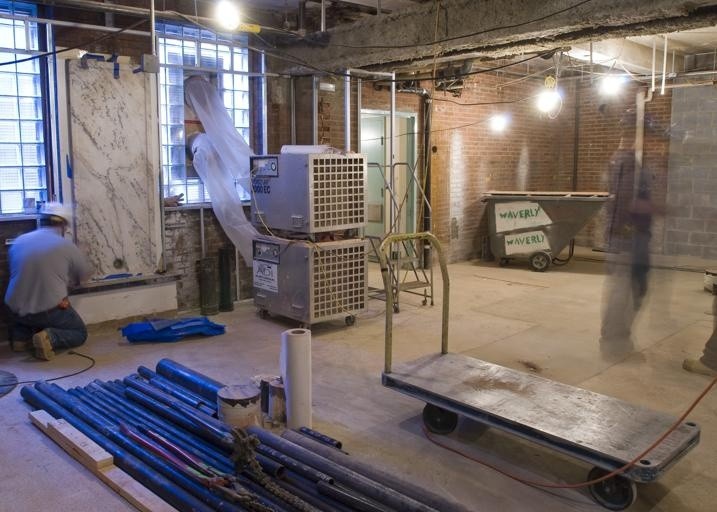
xmin=39 ymin=201 xmax=74 ymax=228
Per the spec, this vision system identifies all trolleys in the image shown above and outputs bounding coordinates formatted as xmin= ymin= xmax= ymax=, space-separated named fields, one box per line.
xmin=378 ymin=229 xmax=701 ymax=511
xmin=480 ymin=189 xmax=616 ymax=272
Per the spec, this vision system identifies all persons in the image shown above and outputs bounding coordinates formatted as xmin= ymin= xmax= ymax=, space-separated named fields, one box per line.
xmin=680 ymin=324 xmax=716 ymax=380
xmin=1 ymin=200 xmax=94 ymax=361
xmin=593 ymin=108 xmax=677 ymax=366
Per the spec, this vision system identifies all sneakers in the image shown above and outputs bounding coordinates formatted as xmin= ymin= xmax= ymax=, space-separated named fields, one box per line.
xmin=682 ymin=357 xmax=717 ymax=377
xmin=33 ymin=330 xmax=55 ymax=362
xmin=11 ymin=340 xmax=29 ymax=352
